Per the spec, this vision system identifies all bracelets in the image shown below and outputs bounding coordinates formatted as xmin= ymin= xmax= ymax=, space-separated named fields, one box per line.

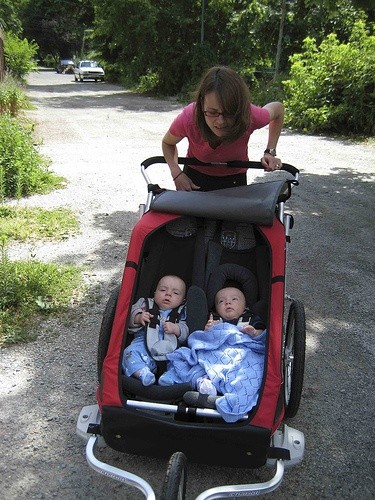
xmin=173 ymin=171 xmax=184 ymax=181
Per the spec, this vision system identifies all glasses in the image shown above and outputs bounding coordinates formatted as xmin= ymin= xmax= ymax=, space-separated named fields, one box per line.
xmin=201 ymin=99 xmax=235 ymax=118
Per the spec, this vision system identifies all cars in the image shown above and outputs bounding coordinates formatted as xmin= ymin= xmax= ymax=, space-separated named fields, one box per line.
xmin=57 ymin=59 xmax=75 ymax=74
xmin=75 ymin=61 xmax=105 ymax=81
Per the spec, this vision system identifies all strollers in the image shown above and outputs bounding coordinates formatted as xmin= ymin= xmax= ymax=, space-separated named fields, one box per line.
xmin=76 ymin=156 xmax=306 ymax=500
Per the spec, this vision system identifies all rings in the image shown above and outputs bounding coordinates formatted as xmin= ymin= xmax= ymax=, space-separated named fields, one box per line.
xmin=275 ymin=165 xmax=279 ymax=168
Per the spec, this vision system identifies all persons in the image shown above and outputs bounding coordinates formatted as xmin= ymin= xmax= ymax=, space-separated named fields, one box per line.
xmin=121 ymin=274 xmax=190 ymax=387
xmin=161 ymin=66 xmax=285 ymax=192
xmin=188 ymin=285 xmax=267 ymax=396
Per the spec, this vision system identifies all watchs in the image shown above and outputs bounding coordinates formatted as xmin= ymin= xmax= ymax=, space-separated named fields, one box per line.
xmin=264 ymin=148 xmax=276 ymax=156
